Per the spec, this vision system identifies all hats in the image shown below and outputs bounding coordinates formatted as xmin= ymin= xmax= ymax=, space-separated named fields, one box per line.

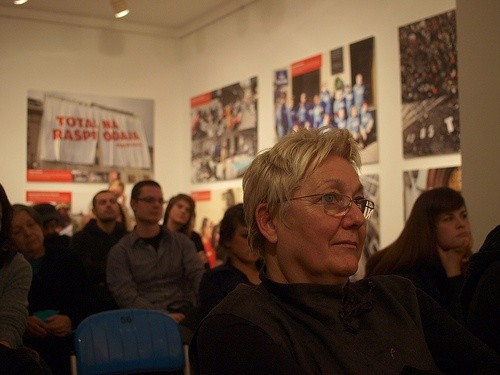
xmin=35 ymin=204 xmax=60 ymax=221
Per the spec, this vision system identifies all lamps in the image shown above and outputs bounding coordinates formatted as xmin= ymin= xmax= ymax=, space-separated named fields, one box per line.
xmin=110 ymin=1 xmax=132 ymax=20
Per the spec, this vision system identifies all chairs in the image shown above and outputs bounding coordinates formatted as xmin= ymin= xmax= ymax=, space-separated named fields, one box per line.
xmin=70 ymin=309 xmax=190 ymax=374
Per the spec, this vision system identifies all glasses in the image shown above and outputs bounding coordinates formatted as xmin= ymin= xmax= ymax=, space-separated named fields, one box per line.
xmin=277 ymin=191 xmax=375 ymax=220
xmin=137 ymin=197 xmax=165 ymax=205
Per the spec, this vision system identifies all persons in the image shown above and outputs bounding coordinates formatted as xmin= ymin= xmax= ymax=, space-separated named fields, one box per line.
xmin=276 ymin=73 xmax=374 ymax=149
xmin=0 ymin=124 xmax=500 ymax=375
